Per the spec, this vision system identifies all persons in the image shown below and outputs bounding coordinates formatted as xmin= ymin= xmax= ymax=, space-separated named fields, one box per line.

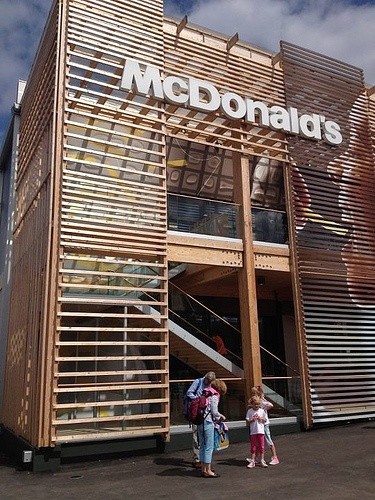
xmin=197 ymin=380 xmax=227 ymax=478
xmin=208 ymin=331 xmax=228 ymax=355
xmin=289 ymin=92 xmax=375 ymax=309
xmin=186 ymin=371 xmax=216 ymax=468
xmin=245 ymin=386 xmax=279 ymax=469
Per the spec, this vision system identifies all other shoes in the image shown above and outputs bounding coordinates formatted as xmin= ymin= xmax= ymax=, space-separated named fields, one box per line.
xmin=192 ymin=462 xmax=202 ymax=468
xmin=200 ymin=472 xmax=220 ymax=478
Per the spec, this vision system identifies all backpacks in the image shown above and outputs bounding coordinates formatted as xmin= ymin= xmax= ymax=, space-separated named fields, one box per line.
xmin=183 ymin=378 xmax=201 ymax=419
xmin=188 ymin=390 xmax=216 ymax=425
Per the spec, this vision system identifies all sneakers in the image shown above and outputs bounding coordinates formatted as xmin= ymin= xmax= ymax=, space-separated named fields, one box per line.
xmin=269 ymin=457 xmax=279 ymax=465
xmin=259 ymin=459 xmax=268 ymax=468
xmin=247 ymin=460 xmax=255 ymax=469
xmin=246 ymin=457 xmax=259 ymax=463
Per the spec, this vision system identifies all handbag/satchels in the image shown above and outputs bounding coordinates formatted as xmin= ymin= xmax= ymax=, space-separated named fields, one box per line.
xmin=214 ymin=420 xmax=230 ymax=451
